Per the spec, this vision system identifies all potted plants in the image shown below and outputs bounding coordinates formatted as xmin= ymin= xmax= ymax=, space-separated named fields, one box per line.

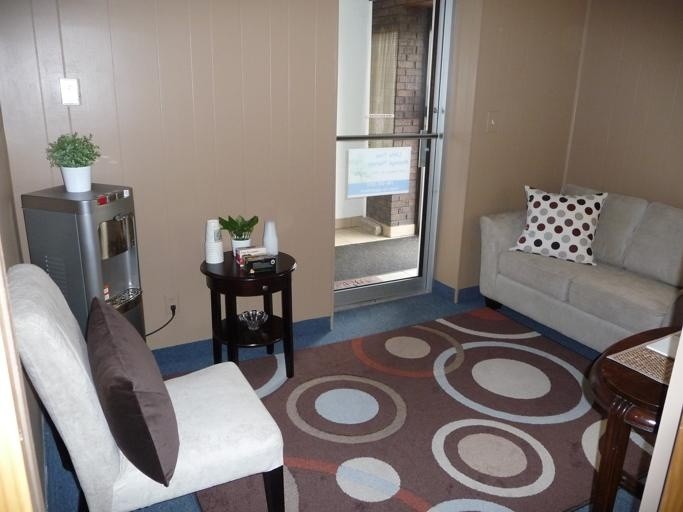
xmin=218 ymin=215 xmax=259 ymax=257
xmin=46 ymin=131 xmax=102 ymax=193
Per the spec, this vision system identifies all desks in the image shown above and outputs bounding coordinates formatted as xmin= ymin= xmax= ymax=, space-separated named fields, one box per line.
xmin=587 ymin=323 xmax=683 ymax=512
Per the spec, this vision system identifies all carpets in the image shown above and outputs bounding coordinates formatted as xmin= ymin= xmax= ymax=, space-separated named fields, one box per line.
xmin=162 ymin=305 xmax=656 ymax=512
xmin=334 ymin=235 xmax=419 ymax=282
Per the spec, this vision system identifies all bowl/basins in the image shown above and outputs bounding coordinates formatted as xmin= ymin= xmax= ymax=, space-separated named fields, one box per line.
xmin=239 ymin=310 xmax=269 ymax=331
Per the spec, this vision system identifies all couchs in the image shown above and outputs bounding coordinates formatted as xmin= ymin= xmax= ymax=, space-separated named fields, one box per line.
xmin=478 ymin=183 xmax=683 ymax=362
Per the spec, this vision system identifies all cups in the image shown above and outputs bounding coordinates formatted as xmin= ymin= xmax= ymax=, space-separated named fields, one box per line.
xmin=262 ymin=221 xmax=278 ymax=256
xmin=204 ymin=219 xmax=224 ymax=265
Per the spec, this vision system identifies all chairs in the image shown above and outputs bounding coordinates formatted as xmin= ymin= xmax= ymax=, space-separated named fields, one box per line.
xmin=5 ymin=262 xmax=285 ymax=511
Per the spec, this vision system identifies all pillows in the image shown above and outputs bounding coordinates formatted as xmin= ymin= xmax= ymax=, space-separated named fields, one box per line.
xmin=85 ymin=296 xmax=181 ymax=488
xmin=508 ymin=185 xmax=608 ymax=267
